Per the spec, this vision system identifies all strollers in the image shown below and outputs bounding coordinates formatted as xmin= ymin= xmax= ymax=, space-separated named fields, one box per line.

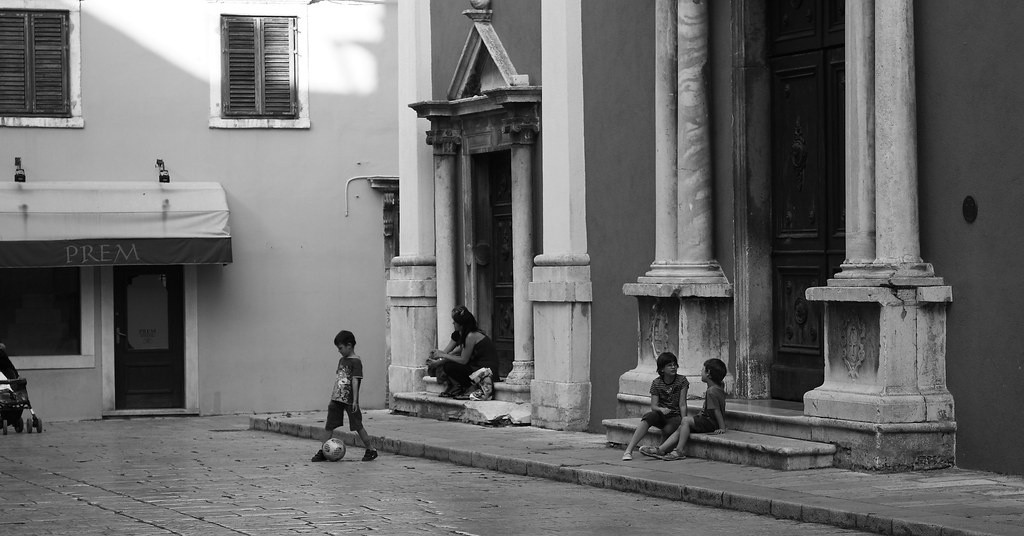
xmin=0 ymin=343 xmax=43 ymax=436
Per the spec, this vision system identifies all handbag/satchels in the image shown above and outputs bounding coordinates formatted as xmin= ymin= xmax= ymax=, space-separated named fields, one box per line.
xmin=469 ymin=367 xmax=494 ymax=401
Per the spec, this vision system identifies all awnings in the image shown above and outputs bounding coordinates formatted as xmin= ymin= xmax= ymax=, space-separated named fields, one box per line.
xmin=0 ymin=182 xmax=233 ymax=269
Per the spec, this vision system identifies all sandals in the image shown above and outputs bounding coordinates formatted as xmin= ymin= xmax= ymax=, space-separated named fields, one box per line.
xmin=662 ymin=449 xmax=686 ymax=461
xmin=639 ymin=445 xmax=667 ymax=459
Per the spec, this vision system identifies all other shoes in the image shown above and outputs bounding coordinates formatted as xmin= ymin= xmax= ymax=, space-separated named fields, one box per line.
xmin=439 ymin=390 xmax=453 ymax=397
xmin=312 ymin=448 xmax=327 ymax=462
xmin=362 ymin=449 xmax=378 ymax=462
xmin=623 ymin=451 xmax=632 ymax=460
xmin=454 ymin=394 xmax=470 ymax=400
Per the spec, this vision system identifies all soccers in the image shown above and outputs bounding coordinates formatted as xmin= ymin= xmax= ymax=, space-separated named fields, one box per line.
xmin=321 ymin=437 xmax=347 ymax=461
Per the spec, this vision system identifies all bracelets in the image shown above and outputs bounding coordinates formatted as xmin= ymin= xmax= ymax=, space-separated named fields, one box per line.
xmin=439 ymin=357 xmax=442 ymax=360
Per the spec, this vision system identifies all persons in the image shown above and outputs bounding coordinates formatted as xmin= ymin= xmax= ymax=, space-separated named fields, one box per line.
xmin=311 ymin=331 xmax=378 ymax=462
xmin=622 ymin=353 xmax=690 ymax=460
xmin=639 ymin=358 xmax=727 ymax=461
xmin=428 ymin=306 xmax=500 ymax=398
